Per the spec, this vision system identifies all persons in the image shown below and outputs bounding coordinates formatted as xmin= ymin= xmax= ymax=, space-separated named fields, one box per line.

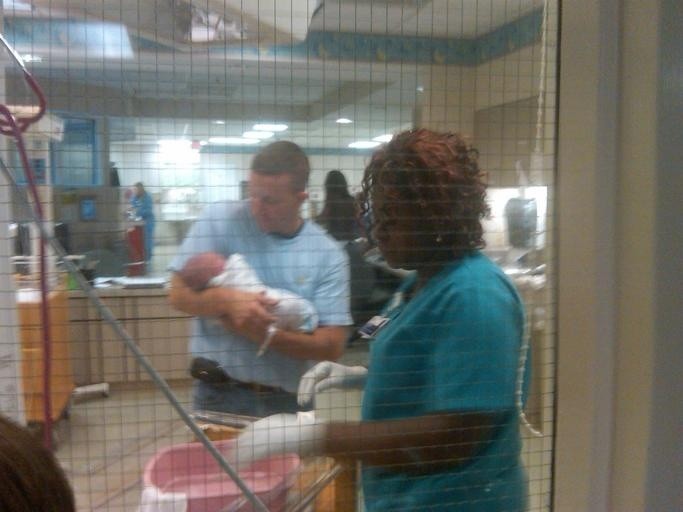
xmin=163 ymin=139 xmax=353 ymax=424
xmin=226 ymin=127 xmax=532 ymax=511
xmin=0 ymin=415 xmax=78 ymax=512
xmin=175 ymin=249 xmax=319 ymax=335
xmin=314 ymin=165 xmax=366 ymax=345
xmin=126 ymin=180 xmax=158 ymax=274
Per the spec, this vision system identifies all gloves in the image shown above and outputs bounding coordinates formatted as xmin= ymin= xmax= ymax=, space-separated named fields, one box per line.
xmin=231 ymin=414 xmax=320 ymax=472
xmin=296 ymin=360 xmax=368 ymax=405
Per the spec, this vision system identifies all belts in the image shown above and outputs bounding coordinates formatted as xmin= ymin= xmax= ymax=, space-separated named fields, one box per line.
xmin=228 ymin=379 xmax=277 ymax=392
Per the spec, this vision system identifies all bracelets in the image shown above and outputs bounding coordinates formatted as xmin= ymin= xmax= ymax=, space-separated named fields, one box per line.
xmin=256 ymin=325 xmax=275 ymax=358
